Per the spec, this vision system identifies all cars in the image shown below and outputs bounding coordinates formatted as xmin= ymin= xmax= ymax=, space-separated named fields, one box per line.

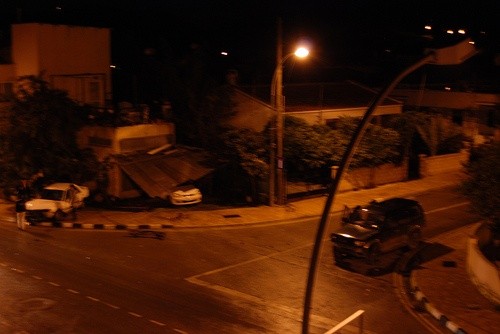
xmin=169 ymin=185 xmax=203 ymax=205
xmin=24 ymin=183 xmax=90 ymax=220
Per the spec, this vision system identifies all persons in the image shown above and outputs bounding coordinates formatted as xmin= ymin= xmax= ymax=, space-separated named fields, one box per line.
xmin=11 ymin=186 xmax=26 ymax=231
xmin=341 ymin=200 xmax=377 ymax=225
xmin=70 ymin=184 xmax=78 ymax=220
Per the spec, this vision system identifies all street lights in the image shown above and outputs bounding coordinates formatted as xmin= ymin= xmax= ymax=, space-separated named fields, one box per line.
xmin=270 ymin=45 xmax=308 ymax=206
xmin=301 ymin=38 xmax=475 ymax=334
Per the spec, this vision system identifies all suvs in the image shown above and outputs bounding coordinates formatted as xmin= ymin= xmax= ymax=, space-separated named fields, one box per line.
xmin=331 ymin=198 xmax=428 ymax=258
